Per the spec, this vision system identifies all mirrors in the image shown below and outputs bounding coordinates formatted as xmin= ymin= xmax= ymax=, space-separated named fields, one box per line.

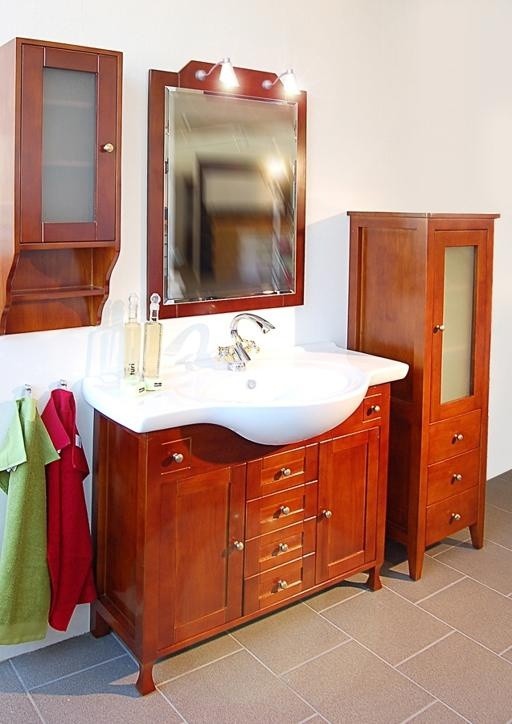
xmin=146 ymin=62 xmax=311 ymax=318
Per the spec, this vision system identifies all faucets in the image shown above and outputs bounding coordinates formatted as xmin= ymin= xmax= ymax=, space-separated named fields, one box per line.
xmin=229 ymin=313 xmax=278 ymax=369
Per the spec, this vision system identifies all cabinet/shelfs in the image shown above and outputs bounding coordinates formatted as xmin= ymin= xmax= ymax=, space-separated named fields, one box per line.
xmin=1 ymin=36 xmax=122 ymax=339
xmin=90 ymin=384 xmax=390 ymax=659
xmin=348 ymin=208 xmax=502 ymax=584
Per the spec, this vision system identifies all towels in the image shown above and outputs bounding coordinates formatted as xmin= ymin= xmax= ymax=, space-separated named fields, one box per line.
xmin=1 ymin=389 xmax=101 ymax=645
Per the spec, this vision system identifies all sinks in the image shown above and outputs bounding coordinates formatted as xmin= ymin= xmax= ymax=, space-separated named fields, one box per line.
xmin=175 ymin=353 xmax=371 ymax=447
